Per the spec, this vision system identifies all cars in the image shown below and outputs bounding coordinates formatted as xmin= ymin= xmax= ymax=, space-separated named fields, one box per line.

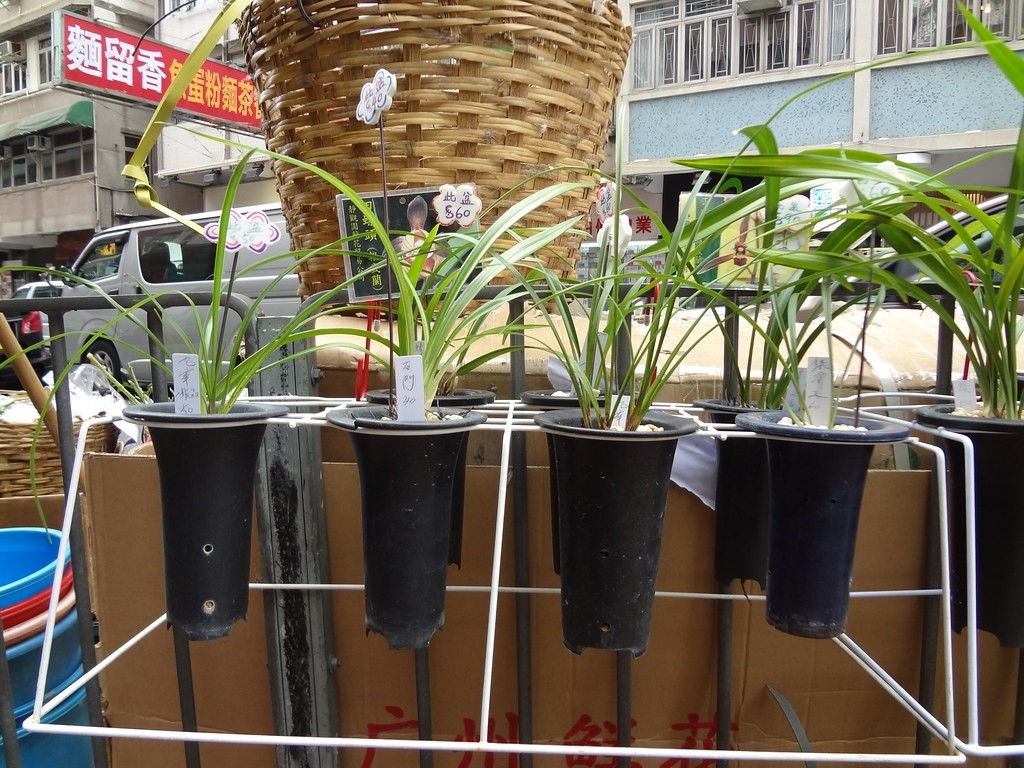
xmin=0 ymin=281 xmax=66 ymax=391
xmin=818 ymin=193 xmax=1024 ymax=310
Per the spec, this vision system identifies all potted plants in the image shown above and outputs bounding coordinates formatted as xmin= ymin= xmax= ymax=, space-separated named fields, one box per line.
xmin=0 ymin=0 xmax=1024 ymax=661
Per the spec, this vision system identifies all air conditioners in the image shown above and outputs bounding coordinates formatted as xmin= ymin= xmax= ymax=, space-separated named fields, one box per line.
xmin=26 ymin=135 xmax=52 ymax=153
xmin=0 ymin=146 xmax=15 ymax=162
xmin=0 ymin=40 xmax=26 ymax=63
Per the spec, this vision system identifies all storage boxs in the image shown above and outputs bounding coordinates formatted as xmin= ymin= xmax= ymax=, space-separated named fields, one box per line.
xmin=81 ymin=428 xmax=1022 ymax=768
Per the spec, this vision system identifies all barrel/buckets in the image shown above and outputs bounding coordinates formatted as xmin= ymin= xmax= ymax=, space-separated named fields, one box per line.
xmin=0 ymin=527 xmax=93 ymax=768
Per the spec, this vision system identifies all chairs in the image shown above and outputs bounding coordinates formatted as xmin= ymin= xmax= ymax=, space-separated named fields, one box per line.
xmin=140 ymin=242 xmax=178 ymax=283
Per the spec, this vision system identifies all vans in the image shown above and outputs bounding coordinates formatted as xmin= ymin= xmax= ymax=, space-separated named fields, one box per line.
xmin=37 ymin=201 xmax=302 ymax=394
